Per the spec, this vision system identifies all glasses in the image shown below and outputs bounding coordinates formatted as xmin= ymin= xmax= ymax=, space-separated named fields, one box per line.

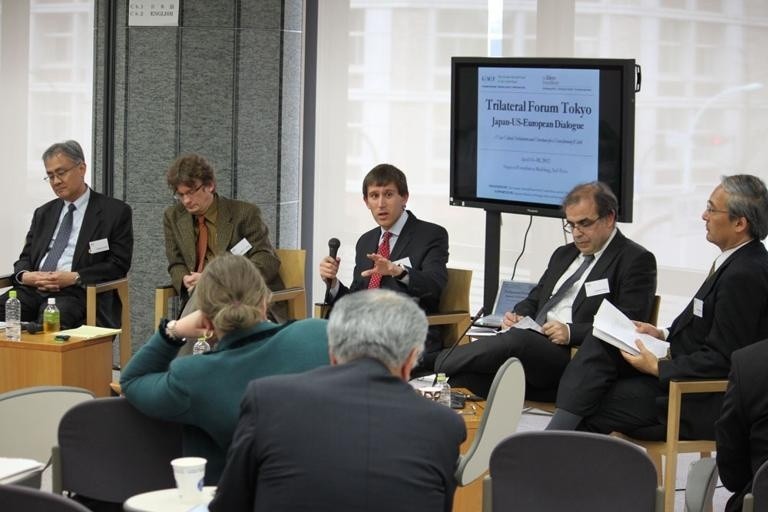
xmin=171 ymin=183 xmax=207 ymax=200
xmin=43 ymin=161 xmax=82 ymax=181
xmin=705 ymin=201 xmax=733 ymax=214
xmin=561 ymin=212 xmax=609 ymax=233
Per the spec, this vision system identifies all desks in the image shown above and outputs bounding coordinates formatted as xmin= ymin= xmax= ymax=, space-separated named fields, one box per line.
xmin=0 ymin=322 xmax=122 ymax=401
xmin=123 ymin=486 xmax=218 ymax=512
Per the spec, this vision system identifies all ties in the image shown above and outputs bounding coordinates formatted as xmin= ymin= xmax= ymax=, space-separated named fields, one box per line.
xmin=196 ymin=214 xmax=209 ymax=273
xmin=40 ymin=203 xmax=77 ymax=272
xmin=366 ymin=230 xmax=393 ymax=289
xmin=533 ymin=253 xmax=595 ymax=327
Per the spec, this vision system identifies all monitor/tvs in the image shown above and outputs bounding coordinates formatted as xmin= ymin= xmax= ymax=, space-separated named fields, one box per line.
xmin=449 ymin=56 xmax=636 ymax=224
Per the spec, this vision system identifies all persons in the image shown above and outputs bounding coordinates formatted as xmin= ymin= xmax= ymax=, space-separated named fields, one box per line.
xmin=161 ymin=156 xmax=288 ymax=355
xmin=208 ymin=289 xmax=465 ymax=512
xmin=421 ymin=179 xmax=657 ymax=396
xmin=0 ymin=140 xmax=133 ymax=328
xmin=120 ymin=254 xmax=328 ymax=488
xmin=544 ymin=174 xmax=768 ymax=443
xmin=713 ymin=336 xmax=768 ymax=512
xmin=320 ymin=163 xmax=448 ymax=367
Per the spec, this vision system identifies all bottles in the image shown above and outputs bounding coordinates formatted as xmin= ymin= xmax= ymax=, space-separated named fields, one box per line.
xmin=192 ymin=337 xmax=210 ymax=355
xmin=433 ymin=372 xmax=451 ymax=408
xmin=43 ymin=298 xmax=60 ymax=335
xmin=5 ymin=291 xmax=20 ymax=340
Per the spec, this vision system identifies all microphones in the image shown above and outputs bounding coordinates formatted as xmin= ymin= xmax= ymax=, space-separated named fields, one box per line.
xmin=324 ymin=238 xmax=340 ymax=299
xmin=431 ymin=308 xmax=486 ymax=386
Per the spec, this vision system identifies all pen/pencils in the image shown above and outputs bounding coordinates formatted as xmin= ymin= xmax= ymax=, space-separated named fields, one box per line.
xmin=477 ymin=403 xmax=484 ymax=409
xmin=513 ymin=312 xmax=517 ymax=323
xmin=472 ymin=404 xmax=476 ymax=410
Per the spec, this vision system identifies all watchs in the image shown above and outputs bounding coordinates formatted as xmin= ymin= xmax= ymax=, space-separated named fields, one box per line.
xmin=166 ymin=319 xmax=183 ymax=340
xmin=75 ymin=271 xmax=81 ymax=285
xmin=395 ymin=264 xmax=409 ymax=280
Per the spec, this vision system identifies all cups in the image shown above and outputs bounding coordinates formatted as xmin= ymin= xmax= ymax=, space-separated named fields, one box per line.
xmin=171 ymin=455 xmax=208 ymax=504
xmin=420 ymin=387 xmax=441 ymax=403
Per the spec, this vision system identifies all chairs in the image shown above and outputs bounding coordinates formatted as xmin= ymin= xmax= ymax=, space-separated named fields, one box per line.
xmin=0 ymin=385 xmax=102 ymax=512
xmin=51 ymin=397 xmax=226 ymax=512
xmin=153 ymin=246 xmax=308 ymax=335
xmin=438 ymin=291 xmax=767 ymax=512
xmin=0 ymin=275 xmax=132 ymax=378
xmin=311 ymin=268 xmax=481 ymax=368
